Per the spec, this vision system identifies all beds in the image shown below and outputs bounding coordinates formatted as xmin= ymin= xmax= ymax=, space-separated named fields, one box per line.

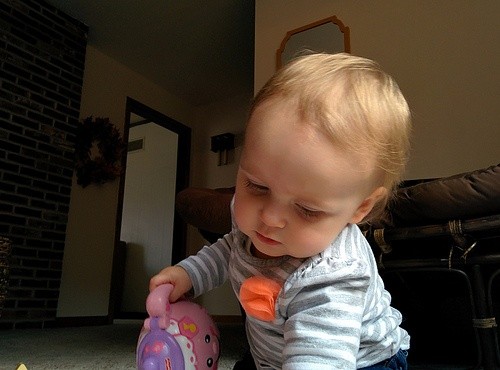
xmin=175 ymin=162 xmax=500 ymax=370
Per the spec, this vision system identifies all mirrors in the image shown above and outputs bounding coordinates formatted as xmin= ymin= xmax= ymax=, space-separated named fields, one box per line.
xmin=276 ymin=15 xmax=351 ymax=71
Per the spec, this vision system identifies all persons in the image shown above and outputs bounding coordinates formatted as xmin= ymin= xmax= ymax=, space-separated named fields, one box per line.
xmin=148 ymin=52 xmax=414 ymax=370
